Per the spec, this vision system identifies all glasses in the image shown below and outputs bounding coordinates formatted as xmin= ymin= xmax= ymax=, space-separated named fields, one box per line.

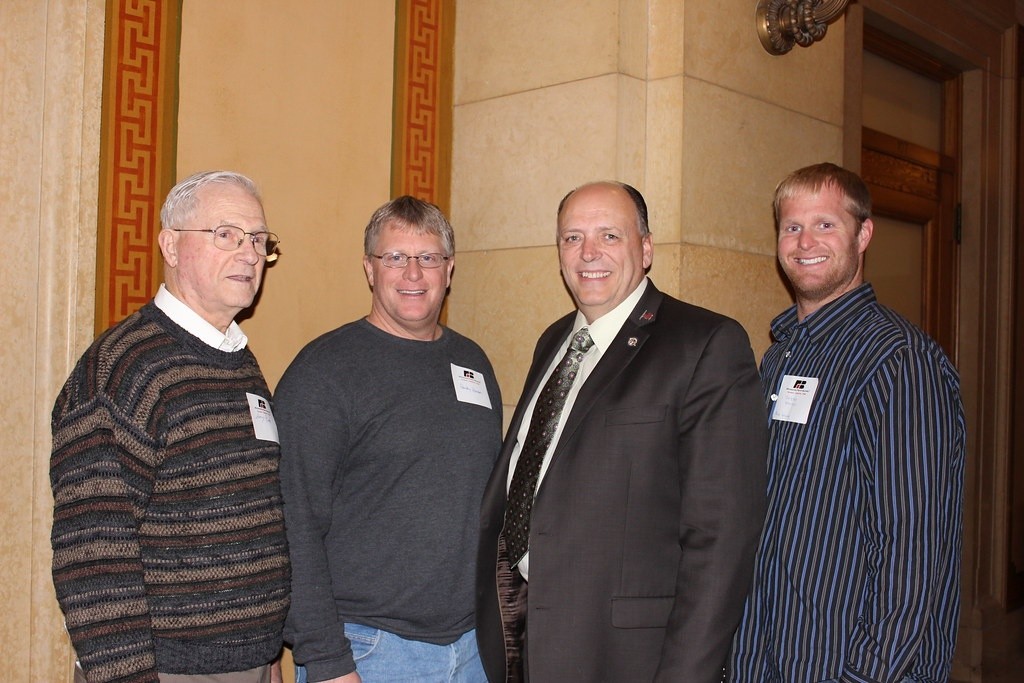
xmin=175 ymin=224 xmax=280 ymax=256
xmin=372 ymin=251 xmax=449 ymax=269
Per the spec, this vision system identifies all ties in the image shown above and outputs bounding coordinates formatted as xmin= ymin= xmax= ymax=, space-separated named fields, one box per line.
xmin=502 ymin=328 xmax=595 ymax=567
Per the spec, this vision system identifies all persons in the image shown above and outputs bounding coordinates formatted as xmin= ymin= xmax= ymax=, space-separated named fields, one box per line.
xmin=475 ymin=179 xmax=768 ymax=682
xmin=273 ymin=194 xmax=503 ymax=683
xmin=723 ymin=161 xmax=967 ymax=683
xmin=49 ymin=172 xmax=293 ymax=683
